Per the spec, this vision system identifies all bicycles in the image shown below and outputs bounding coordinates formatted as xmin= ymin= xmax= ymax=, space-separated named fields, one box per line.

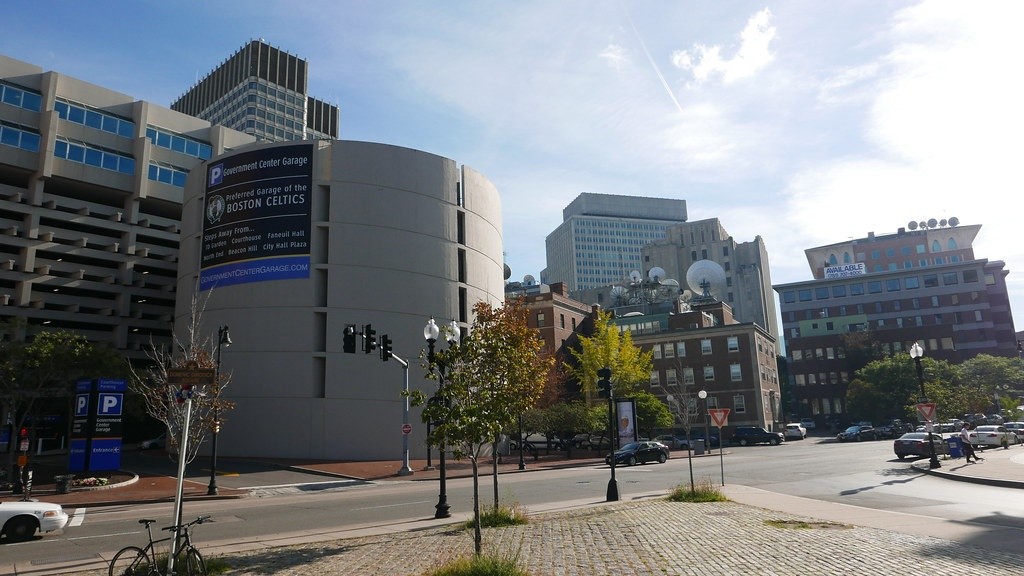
xmin=109 ymin=515 xmax=216 ymax=576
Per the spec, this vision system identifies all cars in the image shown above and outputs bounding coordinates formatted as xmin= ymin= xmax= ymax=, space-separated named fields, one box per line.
xmin=677 ymin=432 xmax=719 ymax=447
xmin=605 ymin=441 xmax=670 ymax=466
xmin=509 ymin=432 xmax=567 ymax=452
xmin=0 ymin=501 xmax=69 ymax=542
xmin=651 ymin=435 xmax=695 ymax=450
xmin=836 ymin=426 xmax=879 ymax=442
xmin=570 ymin=433 xmax=611 ymax=452
xmin=894 ymin=421 xmax=1024 ymax=460
xmin=800 ymin=417 xmax=816 ymax=430
xmin=875 ymin=408 xmax=1016 ymax=440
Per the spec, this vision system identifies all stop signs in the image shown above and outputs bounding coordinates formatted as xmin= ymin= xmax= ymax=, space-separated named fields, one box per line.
xmin=402 ymin=424 xmax=412 ymax=435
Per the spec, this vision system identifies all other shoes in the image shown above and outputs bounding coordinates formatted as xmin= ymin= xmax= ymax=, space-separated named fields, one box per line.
xmin=975 ymin=458 xmax=982 ymax=461
xmin=967 ymin=460 xmax=973 ymax=463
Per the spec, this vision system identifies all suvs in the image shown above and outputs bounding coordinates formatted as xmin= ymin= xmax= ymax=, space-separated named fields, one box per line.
xmin=785 ymin=423 xmax=807 ymax=440
xmin=732 ymin=426 xmax=785 ymax=447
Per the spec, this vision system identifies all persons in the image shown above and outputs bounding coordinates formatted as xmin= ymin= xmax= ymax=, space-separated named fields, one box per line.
xmin=961 ymin=424 xmax=982 ymax=463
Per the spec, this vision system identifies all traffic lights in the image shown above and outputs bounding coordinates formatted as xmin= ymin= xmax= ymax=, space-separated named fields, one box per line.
xmin=382 ymin=334 xmax=393 ymax=361
xmin=18 ymin=428 xmax=33 ymax=438
xmin=365 ymin=324 xmax=376 ymax=353
xmin=342 ymin=325 xmax=355 ymax=354
xmin=597 ymin=369 xmax=611 ymax=398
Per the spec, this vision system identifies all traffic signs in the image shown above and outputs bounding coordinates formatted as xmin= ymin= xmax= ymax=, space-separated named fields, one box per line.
xmin=917 ymin=396 xmax=927 ymax=403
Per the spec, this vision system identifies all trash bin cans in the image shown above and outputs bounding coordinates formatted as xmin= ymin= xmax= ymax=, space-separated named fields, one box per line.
xmin=694 ymin=439 xmax=704 ymax=455
xmin=947 ymin=437 xmax=964 ymax=458
xmin=54 ymin=475 xmax=75 ymax=494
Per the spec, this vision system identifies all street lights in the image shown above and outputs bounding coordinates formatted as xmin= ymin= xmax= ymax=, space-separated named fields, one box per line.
xmin=667 ymin=394 xmax=674 ymax=412
xmin=910 ymin=342 xmax=941 ymax=469
xmin=424 ymin=315 xmax=462 ymax=519
xmin=206 ymin=324 xmax=233 ymax=496
xmin=698 ymin=390 xmax=711 ymax=454
